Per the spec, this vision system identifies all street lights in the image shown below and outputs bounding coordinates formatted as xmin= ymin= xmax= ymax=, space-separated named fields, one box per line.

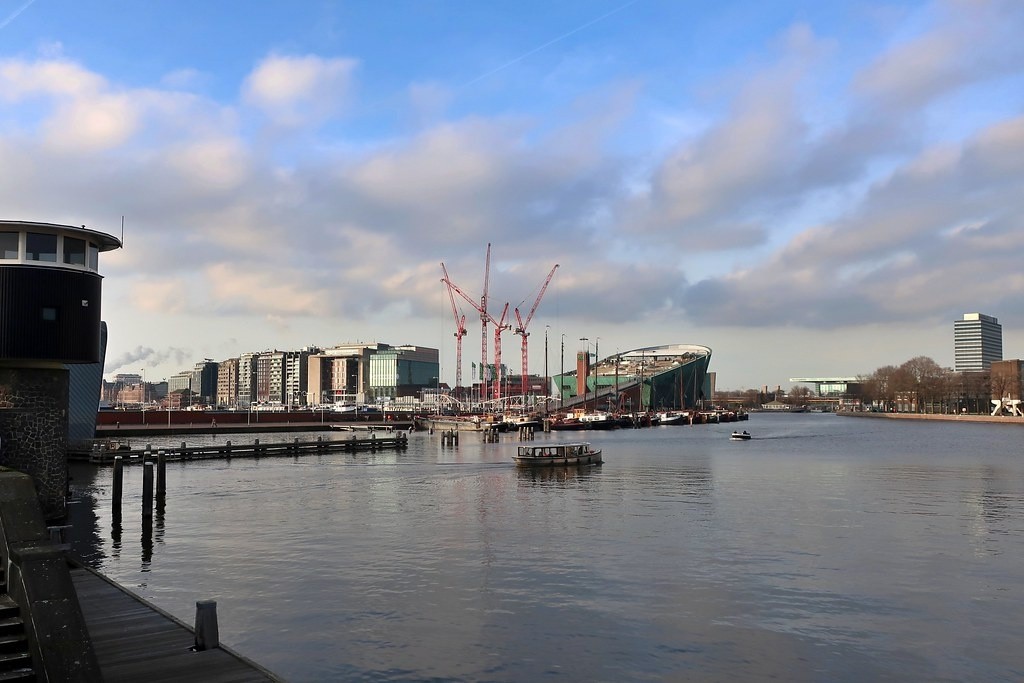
xmin=561 ymin=333 xmax=567 ymax=411
xmin=544 ymin=324 xmax=551 ymax=418
xmin=352 ymin=375 xmax=358 ymax=418
xmin=253 ymin=372 xmax=258 ymax=423
xmin=433 ymin=377 xmax=439 ymax=409
xmin=141 ymin=368 xmax=145 ymax=425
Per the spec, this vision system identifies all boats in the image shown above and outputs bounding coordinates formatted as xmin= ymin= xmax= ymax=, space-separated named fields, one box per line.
xmin=732 ymin=432 xmax=752 ymax=440
xmin=511 ymin=442 xmax=603 ymax=467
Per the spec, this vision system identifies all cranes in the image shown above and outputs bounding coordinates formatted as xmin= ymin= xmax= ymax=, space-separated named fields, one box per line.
xmin=513 ymin=263 xmax=560 ymax=415
xmin=480 ymin=242 xmax=492 ymax=402
xmin=439 ymin=261 xmax=468 ymax=408
xmin=440 ymin=278 xmax=512 ymax=400
